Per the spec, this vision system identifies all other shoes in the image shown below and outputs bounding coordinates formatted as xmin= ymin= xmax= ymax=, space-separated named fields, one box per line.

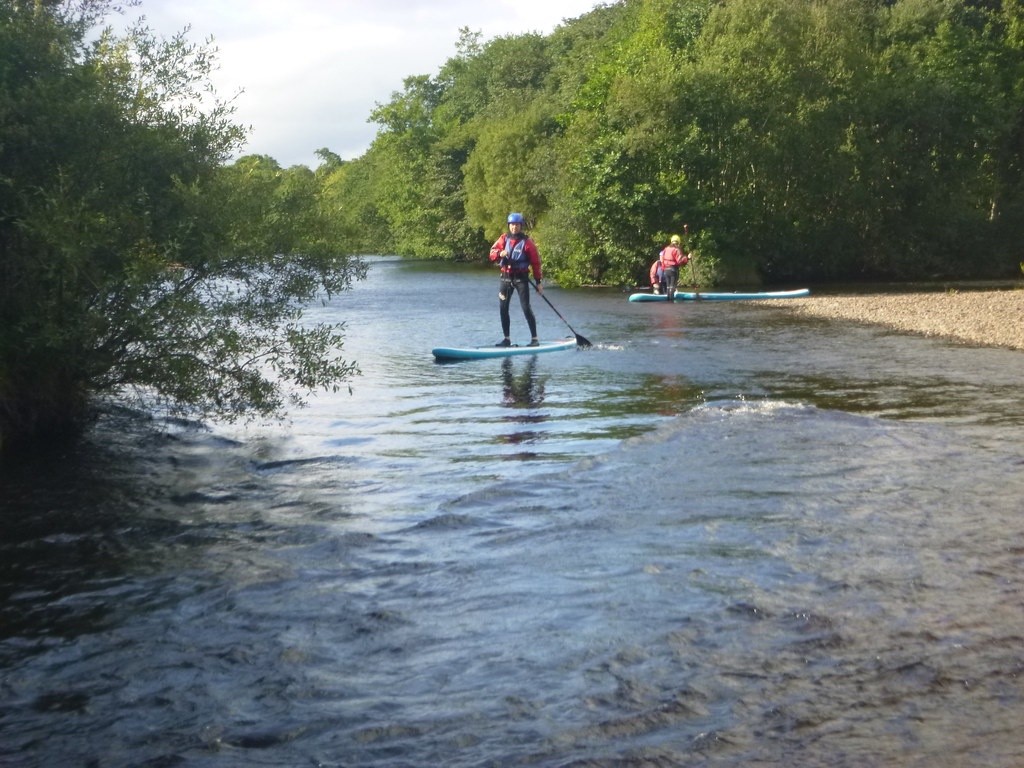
xmin=495 ymin=338 xmax=510 ymax=347
xmin=526 ymin=340 xmax=539 ymax=346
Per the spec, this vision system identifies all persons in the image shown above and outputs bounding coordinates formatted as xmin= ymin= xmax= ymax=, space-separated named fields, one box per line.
xmin=650 ymin=251 xmax=667 ymax=295
xmin=659 ymin=235 xmax=692 ymax=300
xmin=490 ymin=213 xmax=542 ymax=347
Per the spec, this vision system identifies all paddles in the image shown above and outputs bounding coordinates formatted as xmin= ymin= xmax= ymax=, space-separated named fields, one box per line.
xmin=683 ymin=225 xmax=700 ymax=303
xmin=509 ymin=261 xmax=592 ymax=348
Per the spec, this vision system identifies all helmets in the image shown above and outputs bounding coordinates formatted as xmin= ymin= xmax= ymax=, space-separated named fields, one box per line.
xmin=508 ymin=213 xmax=524 ymax=228
xmin=659 ymin=251 xmax=663 ymax=256
xmin=671 ymin=234 xmax=680 ymax=244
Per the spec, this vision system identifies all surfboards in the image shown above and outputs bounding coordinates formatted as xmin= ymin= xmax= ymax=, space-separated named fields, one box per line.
xmin=431 ymin=338 xmax=589 ymax=366
xmin=626 ymin=287 xmax=810 ymax=303
